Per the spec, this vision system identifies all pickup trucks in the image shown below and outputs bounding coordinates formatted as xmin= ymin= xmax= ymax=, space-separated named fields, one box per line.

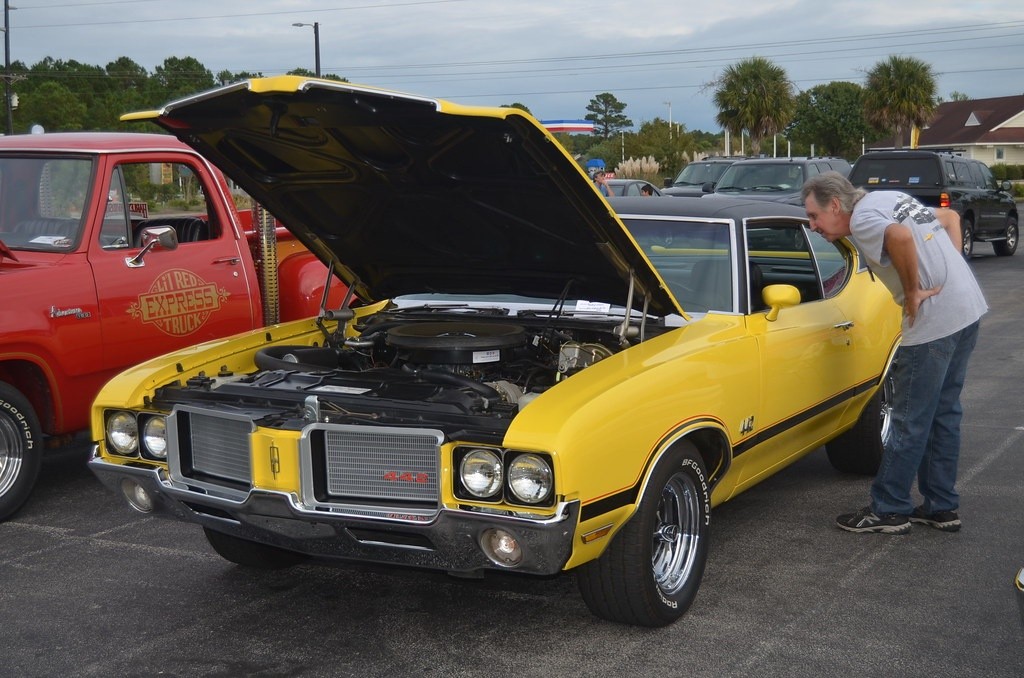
xmin=0 ymin=130 xmax=366 ymax=526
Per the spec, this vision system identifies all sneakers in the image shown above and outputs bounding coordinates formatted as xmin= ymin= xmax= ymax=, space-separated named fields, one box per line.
xmin=907 ymin=504 xmax=963 ymax=533
xmin=836 ymin=506 xmax=911 ymax=534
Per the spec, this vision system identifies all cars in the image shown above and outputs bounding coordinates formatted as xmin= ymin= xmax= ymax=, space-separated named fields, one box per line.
xmin=85 ymin=73 xmax=909 ymax=631
xmin=590 ymin=178 xmax=665 ymax=199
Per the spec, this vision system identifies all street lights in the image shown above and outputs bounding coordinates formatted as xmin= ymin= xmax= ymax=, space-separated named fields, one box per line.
xmin=664 ymin=102 xmax=673 ymax=140
xmin=291 ymin=22 xmax=321 ymax=79
xmin=619 ymin=131 xmax=625 ymax=162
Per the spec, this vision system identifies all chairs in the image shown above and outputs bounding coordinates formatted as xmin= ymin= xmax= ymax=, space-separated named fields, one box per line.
xmin=14 ymin=217 xmax=80 ymax=240
xmin=688 ymin=259 xmax=763 ymax=315
xmin=133 ymin=216 xmax=208 ymax=248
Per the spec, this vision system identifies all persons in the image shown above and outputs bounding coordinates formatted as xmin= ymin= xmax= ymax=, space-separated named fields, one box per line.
xmin=640 ymin=185 xmax=653 ymax=197
xmin=592 ymin=171 xmax=614 ymax=197
xmin=799 ymin=172 xmax=989 ymax=533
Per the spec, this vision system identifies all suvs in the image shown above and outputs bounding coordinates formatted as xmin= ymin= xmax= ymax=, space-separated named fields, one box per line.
xmin=847 ymin=147 xmax=1020 ymax=262
xmin=700 ymin=155 xmax=853 ymax=207
xmin=662 ymin=153 xmax=789 ymax=198
